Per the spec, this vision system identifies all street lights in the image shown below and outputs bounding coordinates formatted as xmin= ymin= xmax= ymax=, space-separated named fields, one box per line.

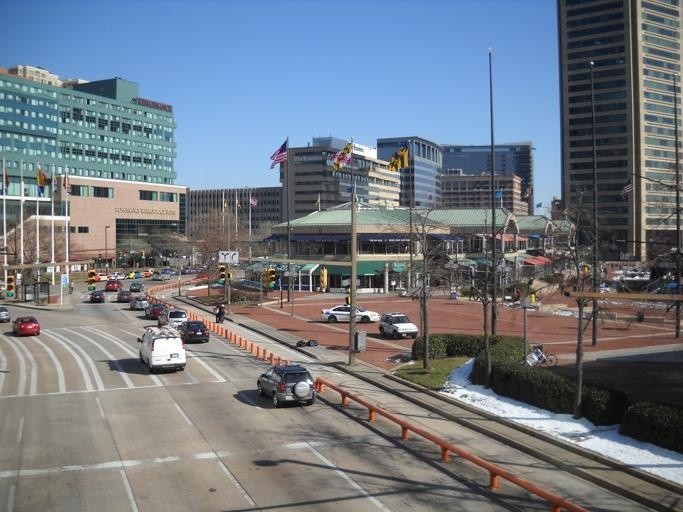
xmin=8 ymin=223 xmax=18 ymax=272
xmin=105 ymin=225 xmax=110 ymax=275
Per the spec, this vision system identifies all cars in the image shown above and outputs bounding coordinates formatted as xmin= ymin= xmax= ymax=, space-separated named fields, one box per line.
xmin=320 ymin=304 xmax=419 ymax=340
xmin=12 ymin=316 xmax=41 ymax=337
xmin=562 ymin=263 xmax=605 ymax=281
xmin=0 ymin=305 xmax=11 ymax=323
xmin=88 ymin=263 xmax=209 ymax=373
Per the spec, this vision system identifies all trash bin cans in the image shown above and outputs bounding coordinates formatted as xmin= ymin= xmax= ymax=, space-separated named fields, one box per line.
xmin=529 ymin=295 xmax=535 ymax=305
xmin=582 ymin=265 xmax=587 ymax=273
xmin=450 ymin=293 xmax=455 ymax=299
xmin=399 ymin=289 xmax=406 ymax=297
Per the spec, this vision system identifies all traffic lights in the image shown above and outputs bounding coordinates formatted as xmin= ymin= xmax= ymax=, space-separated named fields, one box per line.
xmin=319 ymin=266 xmax=330 ymax=292
xmin=228 ymin=272 xmax=232 ymax=280
xmin=263 ymin=267 xmax=276 ymax=288
xmin=218 ymin=265 xmax=226 ymax=284
xmin=5 ymin=275 xmax=16 ymax=298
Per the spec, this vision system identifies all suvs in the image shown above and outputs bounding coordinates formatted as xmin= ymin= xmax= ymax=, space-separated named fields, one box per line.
xmin=257 ymin=363 xmax=317 ymax=408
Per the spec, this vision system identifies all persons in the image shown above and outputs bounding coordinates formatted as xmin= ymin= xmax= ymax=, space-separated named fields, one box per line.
xmin=216 ymin=303 xmax=221 ymax=322
xmin=69 ymin=280 xmax=74 ymax=294
xmin=218 ymin=304 xmax=226 ymax=324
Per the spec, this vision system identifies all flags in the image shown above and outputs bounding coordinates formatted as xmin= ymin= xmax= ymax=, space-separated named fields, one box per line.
xmin=250 ymin=196 xmax=258 ymax=208
xmin=386 ymin=146 xmax=408 ymax=171
xmin=332 ymin=142 xmax=353 ymax=171
xmin=3 ymin=165 xmax=72 ymax=193
xmin=494 ymin=191 xmax=502 ymax=199
xmin=236 ymin=199 xmax=241 ymax=208
xmin=536 ymin=203 xmax=542 ymax=208
xmin=346 ymin=186 xmax=356 ymax=193
xmin=270 ymin=139 xmax=287 ymax=170
xmin=224 ymin=201 xmax=228 ymax=208
xmin=623 ymin=183 xmax=633 ymax=194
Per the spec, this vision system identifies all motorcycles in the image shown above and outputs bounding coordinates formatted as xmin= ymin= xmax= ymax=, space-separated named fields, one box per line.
xmin=532 ymin=344 xmax=558 ymax=367
xmin=216 ymin=303 xmax=226 ymax=324
